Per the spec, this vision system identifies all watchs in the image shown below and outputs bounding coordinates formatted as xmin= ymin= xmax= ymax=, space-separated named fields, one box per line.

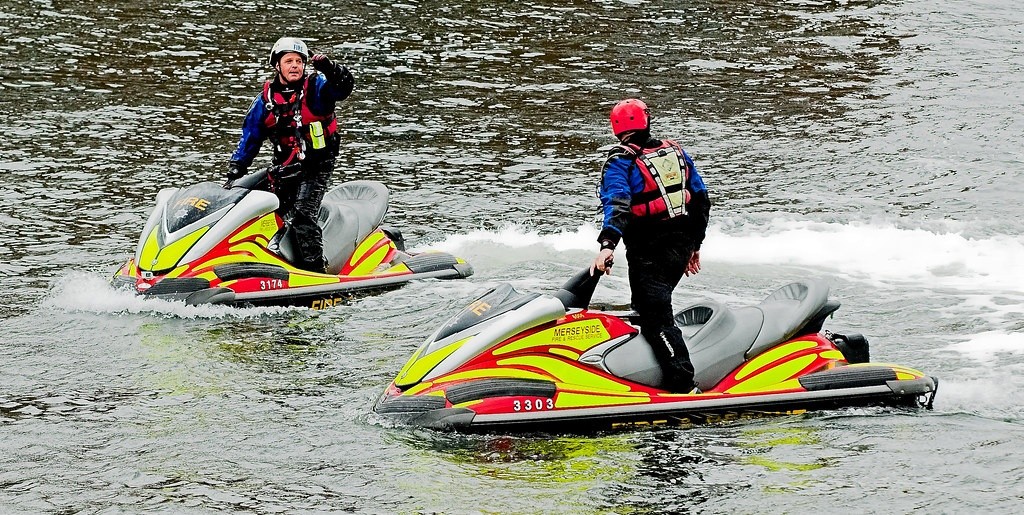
xmin=600 ymin=241 xmax=615 ymax=250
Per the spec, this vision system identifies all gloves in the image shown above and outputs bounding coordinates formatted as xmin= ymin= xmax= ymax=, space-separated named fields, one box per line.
xmin=307 ymin=49 xmax=336 ymax=76
xmin=223 ymin=160 xmax=248 ymax=190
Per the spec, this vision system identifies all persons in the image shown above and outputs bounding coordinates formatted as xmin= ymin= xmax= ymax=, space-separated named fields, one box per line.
xmin=589 ymin=99 xmax=711 ymax=394
xmin=225 ymin=36 xmax=355 ymax=275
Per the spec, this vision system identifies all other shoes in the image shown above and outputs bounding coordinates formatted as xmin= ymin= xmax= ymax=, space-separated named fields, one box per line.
xmin=671 ymin=381 xmax=703 ymax=394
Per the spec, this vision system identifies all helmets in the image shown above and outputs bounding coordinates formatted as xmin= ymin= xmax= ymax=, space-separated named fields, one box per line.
xmin=611 ymin=98 xmax=652 ymax=142
xmin=268 ymin=36 xmax=308 ymax=69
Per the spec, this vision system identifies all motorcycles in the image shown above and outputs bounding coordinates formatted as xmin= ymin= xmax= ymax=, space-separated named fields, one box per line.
xmin=371 ymin=253 xmax=940 ymax=439
xmin=106 ymin=160 xmax=473 ymax=306
